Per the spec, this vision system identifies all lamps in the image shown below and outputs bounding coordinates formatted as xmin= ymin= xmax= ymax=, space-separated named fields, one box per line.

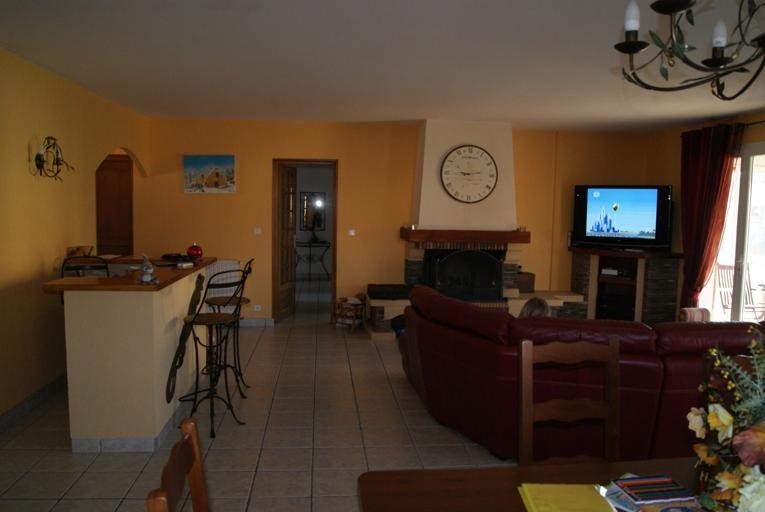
xmin=33 ymin=136 xmax=80 ymax=183
xmin=611 ymin=0 xmax=765 ymax=101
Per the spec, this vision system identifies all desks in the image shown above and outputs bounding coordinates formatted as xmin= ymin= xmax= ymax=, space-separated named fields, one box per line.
xmin=358 ymin=452 xmax=764 ymax=511
xmin=293 ymin=241 xmax=332 ymax=279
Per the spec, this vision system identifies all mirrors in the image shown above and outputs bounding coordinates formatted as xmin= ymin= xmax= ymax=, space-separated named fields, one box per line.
xmin=300 ymin=191 xmax=326 ymax=231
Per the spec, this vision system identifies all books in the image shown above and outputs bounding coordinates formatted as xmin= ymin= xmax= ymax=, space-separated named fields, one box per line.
xmin=604 ymin=472 xmax=705 ymax=512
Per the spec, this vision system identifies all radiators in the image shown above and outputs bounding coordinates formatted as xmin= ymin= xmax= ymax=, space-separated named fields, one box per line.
xmin=205 ymin=259 xmax=242 ymax=316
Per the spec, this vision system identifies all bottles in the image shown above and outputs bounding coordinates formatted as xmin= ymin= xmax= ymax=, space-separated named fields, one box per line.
xmin=185 ymin=241 xmax=202 ymax=258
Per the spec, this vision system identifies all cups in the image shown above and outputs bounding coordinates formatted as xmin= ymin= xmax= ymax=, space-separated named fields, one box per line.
xmin=519 ymin=223 xmax=526 ymax=233
xmin=409 ymin=224 xmax=417 ymax=231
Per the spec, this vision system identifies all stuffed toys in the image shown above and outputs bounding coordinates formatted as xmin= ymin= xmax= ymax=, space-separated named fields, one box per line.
xmin=128 ymin=252 xmax=160 ymax=286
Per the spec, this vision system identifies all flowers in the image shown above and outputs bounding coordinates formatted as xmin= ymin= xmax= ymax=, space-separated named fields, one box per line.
xmin=688 ymin=318 xmax=765 ymax=512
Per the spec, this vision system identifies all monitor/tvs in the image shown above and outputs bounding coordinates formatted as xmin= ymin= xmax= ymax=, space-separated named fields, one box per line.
xmin=573 ymin=184 xmax=672 ymax=248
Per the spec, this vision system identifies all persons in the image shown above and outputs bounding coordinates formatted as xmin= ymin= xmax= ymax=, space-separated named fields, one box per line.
xmin=519 ymin=295 xmax=551 ymax=318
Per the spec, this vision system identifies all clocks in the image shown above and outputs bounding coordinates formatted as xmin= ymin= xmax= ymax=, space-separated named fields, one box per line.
xmin=439 ymin=145 xmax=499 ymax=203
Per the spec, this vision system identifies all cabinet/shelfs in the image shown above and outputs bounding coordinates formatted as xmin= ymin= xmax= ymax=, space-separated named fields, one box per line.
xmin=566 ymin=245 xmax=685 ymax=322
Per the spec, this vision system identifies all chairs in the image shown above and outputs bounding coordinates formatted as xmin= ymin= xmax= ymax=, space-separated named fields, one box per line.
xmin=329 ymin=293 xmax=368 ymax=335
xmin=176 ymin=269 xmax=248 ymax=438
xmin=148 ymin=420 xmax=213 ymax=511
xmin=207 ymin=257 xmax=256 ymax=399
xmin=514 ymin=334 xmax=620 ymax=464
xmin=716 ymin=260 xmax=764 ymax=324
xmin=59 ymin=256 xmax=110 ymax=304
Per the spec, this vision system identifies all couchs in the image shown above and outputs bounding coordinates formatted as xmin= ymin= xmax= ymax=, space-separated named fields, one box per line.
xmin=394 ymin=279 xmax=764 ymax=459
